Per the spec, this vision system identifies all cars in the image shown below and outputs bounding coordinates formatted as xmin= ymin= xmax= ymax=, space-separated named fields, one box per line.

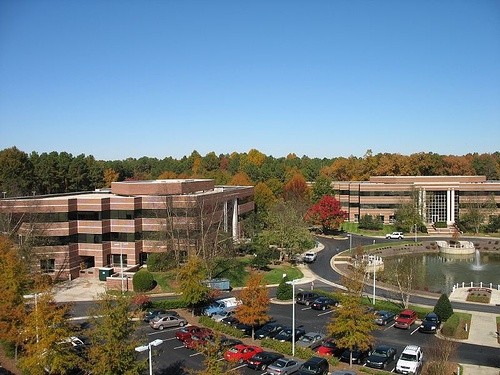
xmin=314 ymin=337 xmax=344 ymax=357
xmin=341 ymin=344 xmax=375 ymax=365
xmin=371 ymin=309 xmax=397 ymax=327
xmin=253 ymin=322 xmax=287 ymax=341
xmin=266 ymin=357 xmax=305 ymax=375
xmin=219 ymin=315 xmax=277 ymax=336
xmin=310 ymin=296 xmax=340 ymax=311
xmin=364 ymin=344 xmax=399 ymax=370
xmin=223 ymin=344 xmax=264 ymax=365
xmin=329 ymin=370 xmax=358 ymax=375
xmin=295 ymin=331 xmax=326 ymax=350
xmin=174 ymin=324 xmax=244 ymax=358
xmin=273 ymin=325 xmax=306 ymax=344
xmin=246 ymin=351 xmax=285 ymax=372
xmin=147 ymin=313 xmax=188 ymax=332
xmin=141 ymin=309 xmax=179 ymax=322
xmin=361 ymin=305 xmax=375 ymax=315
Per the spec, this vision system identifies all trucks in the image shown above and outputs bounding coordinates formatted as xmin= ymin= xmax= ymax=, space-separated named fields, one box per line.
xmin=199 ymin=296 xmax=243 ymax=318
xmin=199 ymin=278 xmax=230 ymax=293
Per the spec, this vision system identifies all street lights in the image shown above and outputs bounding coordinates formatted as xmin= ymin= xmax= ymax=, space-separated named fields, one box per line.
xmin=119 ymin=243 xmax=124 ymax=295
xmin=32 ymin=292 xmax=43 ymax=350
xmin=290 ymin=281 xmax=295 ymax=357
xmin=414 ymin=224 xmax=417 ymax=242
xmin=147 ymin=338 xmax=164 ymax=375
xmin=369 ymin=258 xmax=376 ymax=306
xmin=346 ymin=234 xmax=352 ymax=253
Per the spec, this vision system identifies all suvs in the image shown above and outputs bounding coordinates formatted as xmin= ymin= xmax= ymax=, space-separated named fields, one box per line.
xmin=393 ymin=309 xmax=419 ymax=330
xmin=295 ymin=289 xmax=322 ymax=306
xmin=385 ymin=231 xmax=404 ymax=240
xmin=294 ymin=357 xmax=330 ymax=375
xmin=418 ymin=312 xmax=442 ymax=334
xmin=303 ymin=251 xmax=318 ymax=262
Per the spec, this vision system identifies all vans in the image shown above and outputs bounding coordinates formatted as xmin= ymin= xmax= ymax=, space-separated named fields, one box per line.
xmin=395 ymin=343 xmax=424 ymax=375
xmin=210 ymin=306 xmax=241 ymax=322
